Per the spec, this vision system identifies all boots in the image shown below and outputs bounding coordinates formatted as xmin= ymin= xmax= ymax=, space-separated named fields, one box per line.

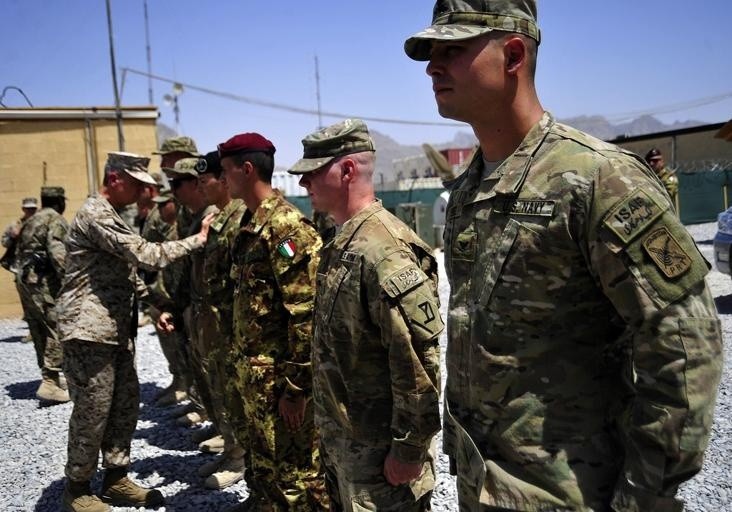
xmin=36 ymin=369 xmax=71 ymax=402
xmin=62 ymin=477 xmax=112 ymax=512
xmin=102 ymin=468 xmax=165 ymax=507
xmin=154 ymin=372 xmax=211 ymax=424
xmin=192 ymin=425 xmax=246 ymax=489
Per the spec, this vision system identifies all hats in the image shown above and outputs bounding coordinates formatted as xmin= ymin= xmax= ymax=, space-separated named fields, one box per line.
xmin=23 ymin=198 xmax=38 ymax=209
xmin=151 ymin=186 xmax=179 ymax=203
xmin=645 ymin=147 xmax=663 ymax=161
xmin=109 ymin=152 xmax=157 ymax=185
xmin=288 ymin=118 xmax=375 ymax=175
xmin=404 ymin=0 xmax=541 ymax=61
xmin=41 ymin=185 xmax=68 ymax=200
xmin=152 ymin=133 xmax=276 ymax=180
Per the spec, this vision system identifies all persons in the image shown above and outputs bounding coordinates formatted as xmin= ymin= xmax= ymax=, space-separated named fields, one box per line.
xmin=403 ymin=0 xmax=725 ymax=512
xmin=1 ymin=117 xmax=445 ymax=511
xmin=643 ymin=147 xmax=679 ymax=213
